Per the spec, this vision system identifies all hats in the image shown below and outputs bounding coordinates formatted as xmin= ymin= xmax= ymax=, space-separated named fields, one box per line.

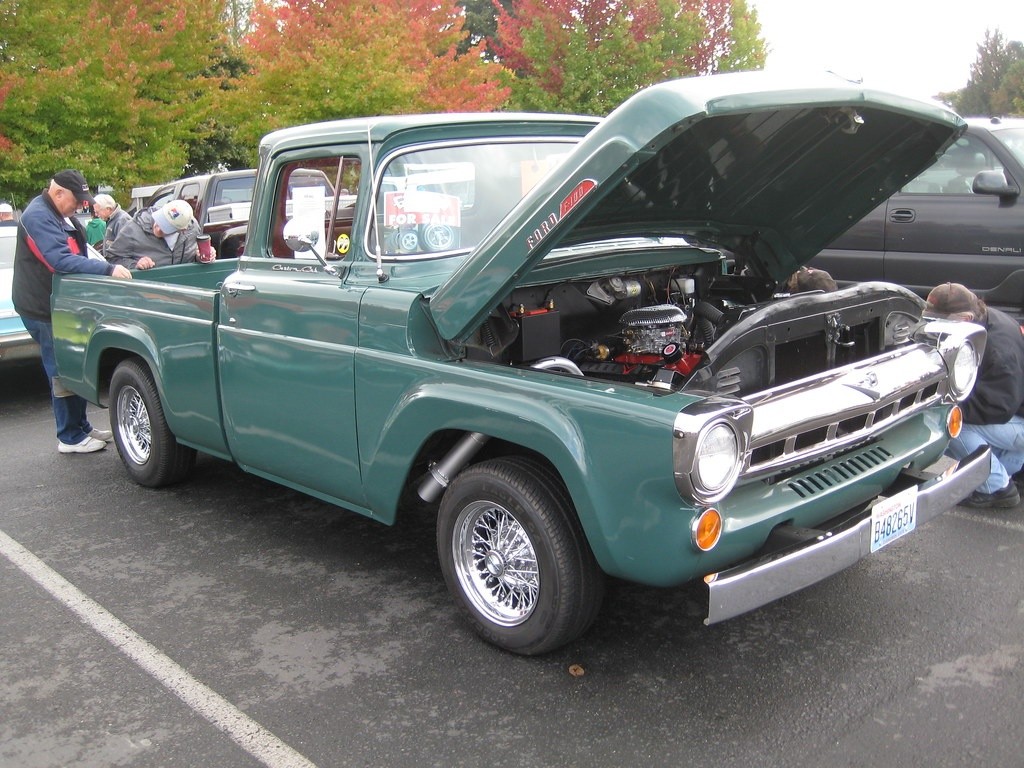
xmin=152 ymin=199 xmax=194 ymax=235
xmin=53 ymin=169 xmax=98 ymax=206
xmin=797 ymin=267 xmax=837 ymax=293
xmin=0 ymin=203 xmax=13 ymax=213
xmin=921 ymin=281 xmax=977 ymax=320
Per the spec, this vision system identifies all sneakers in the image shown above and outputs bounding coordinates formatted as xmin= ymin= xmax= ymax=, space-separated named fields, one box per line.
xmin=958 ymin=479 xmax=1020 ymax=510
xmin=58 ymin=436 xmax=107 ymax=454
xmin=87 ymin=428 xmax=113 ymax=440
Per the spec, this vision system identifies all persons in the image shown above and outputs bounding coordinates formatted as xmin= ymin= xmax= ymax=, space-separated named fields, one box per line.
xmin=772 ymin=266 xmax=838 ymax=295
xmin=922 ymin=282 xmax=1024 ymax=509
xmin=0 ymin=170 xmax=216 ymax=452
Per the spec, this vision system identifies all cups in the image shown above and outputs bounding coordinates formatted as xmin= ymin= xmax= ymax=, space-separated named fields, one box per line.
xmin=196 ymin=234 xmax=211 ymax=263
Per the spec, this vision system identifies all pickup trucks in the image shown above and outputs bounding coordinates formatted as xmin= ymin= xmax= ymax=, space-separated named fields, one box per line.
xmin=1 ymin=71 xmax=1023 ymax=655
